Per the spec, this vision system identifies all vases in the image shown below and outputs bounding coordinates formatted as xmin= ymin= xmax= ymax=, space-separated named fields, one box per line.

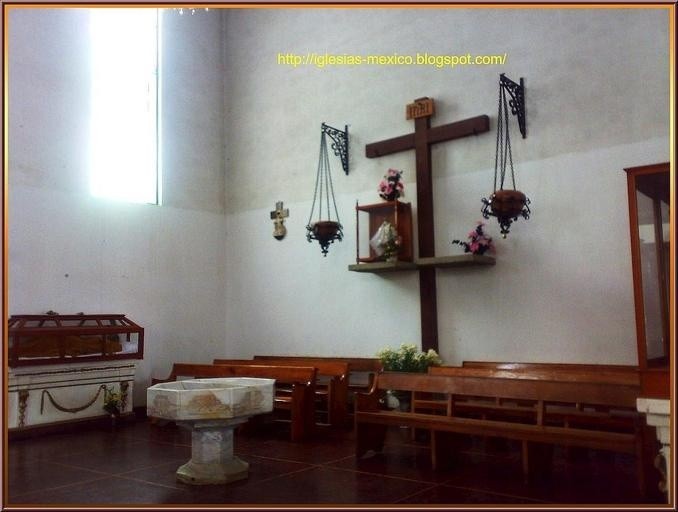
xmin=310 ymin=221 xmax=339 ymax=241
xmin=490 ymin=189 xmax=526 ymax=218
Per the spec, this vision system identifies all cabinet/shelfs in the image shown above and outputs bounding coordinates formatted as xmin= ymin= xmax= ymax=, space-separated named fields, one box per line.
xmin=623 ymin=161 xmax=670 ymax=491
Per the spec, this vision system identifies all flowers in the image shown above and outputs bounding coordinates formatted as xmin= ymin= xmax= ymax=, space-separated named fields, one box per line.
xmin=369 ymin=222 xmax=403 ymax=260
xmin=376 ymin=167 xmax=406 ymax=200
xmin=378 ymin=341 xmax=442 ymax=392
xmin=451 ymin=222 xmax=495 ymax=255
